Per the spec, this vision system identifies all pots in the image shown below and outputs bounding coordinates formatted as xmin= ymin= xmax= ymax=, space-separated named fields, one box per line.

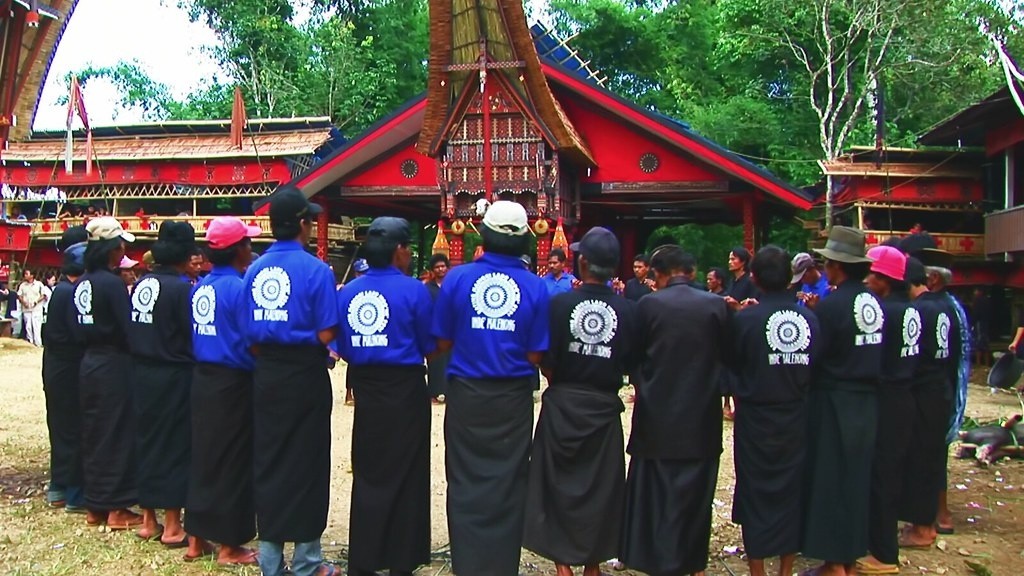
xmin=987 ymin=350 xmax=1024 ymax=390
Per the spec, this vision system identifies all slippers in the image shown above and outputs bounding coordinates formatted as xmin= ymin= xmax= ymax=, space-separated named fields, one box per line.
xmin=313 ymin=565 xmax=340 ymax=576
xmin=106 ymin=514 xmax=144 ymax=529
xmin=183 ymin=543 xmax=219 ymax=561
xmin=48 ymin=502 xmax=65 ymax=508
xmin=136 ymin=525 xmax=189 ymax=548
xmin=86 ymin=515 xmax=105 ymax=526
xmin=64 ymin=504 xmax=88 ymax=514
xmin=217 ymin=548 xmax=259 ymax=566
xmin=793 ymin=522 xmax=954 ymax=576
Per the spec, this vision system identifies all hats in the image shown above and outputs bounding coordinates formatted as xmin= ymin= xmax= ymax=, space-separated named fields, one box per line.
xmin=482 ymin=200 xmax=529 ymax=236
xmin=812 ymin=225 xmax=872 ymax=262
xmin=269 ymin=188 xmax=323 ymax=225
xmin=900 ymin=232 xmax=956 ymax=268
xmin=119 ymin=255 xmax=139 ymax=269
xmin=365 ymin=215 xmax=412 ymax=245
xmin=142 ymin=250 xmax=154 ymax=264
xmin=789 ymin=253 xmax=815 ymax=283
xmin=157 ymin=219 xmax=196 ymax=241
xmin=866 ymin=246 xmax=905 ymax=281
xmin=204 ymin=219 xmax=262 ymax=250
xmin=84 ymin=216 xmax=136 ymax=242
xmin=354 ymin=258 xmax=369 ymax=272
xmin=569 ymin=227 xmax=620 ymax=267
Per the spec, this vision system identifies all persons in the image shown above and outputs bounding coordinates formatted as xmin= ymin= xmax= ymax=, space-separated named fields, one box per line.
xmin=16 ymin=268 xmax=47 ymax=347
xmin=38 ymin=186 xmax=971 ymax=576
xmin=971 ymin=286 xmax=992 ymax=366
xmin=0 ymin=258 xmax=18 ymax=320
xmin=1007 ymin=309 xmax=1024 ymax=354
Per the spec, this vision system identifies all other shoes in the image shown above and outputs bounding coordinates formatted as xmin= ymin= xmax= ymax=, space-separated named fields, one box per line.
xmin=724 ymin=410 xmax=734 ymax=420
xmin=612 ymin=561 xmax=626 ymax=570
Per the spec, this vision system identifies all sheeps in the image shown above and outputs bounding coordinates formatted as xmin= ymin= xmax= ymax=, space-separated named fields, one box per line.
xmin=471 ymin=199 xmax=491 ymax=217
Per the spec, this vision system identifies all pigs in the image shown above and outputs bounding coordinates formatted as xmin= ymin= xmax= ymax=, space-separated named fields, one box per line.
xmin=964 ymin=424 xmax=1024 ymax=467
